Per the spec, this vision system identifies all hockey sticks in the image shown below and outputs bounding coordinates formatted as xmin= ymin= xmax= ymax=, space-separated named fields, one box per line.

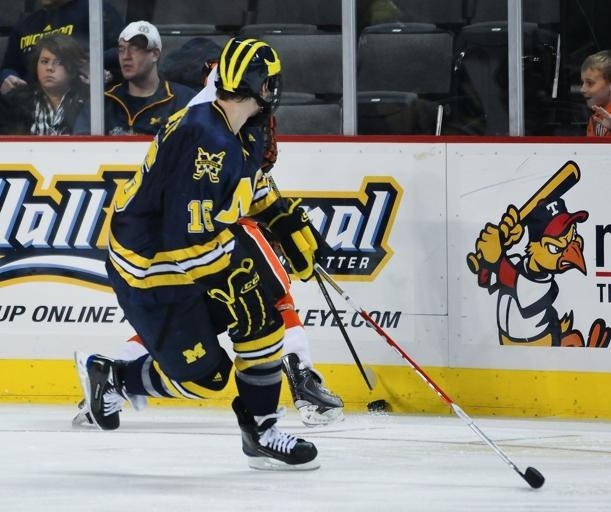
xmin=309 ymin=261 xmax=545 ymax=489
xmin=312 ymin=267 xmax=389 ymax=413
xmin=312 ymin=262 xmax=545 ymax=490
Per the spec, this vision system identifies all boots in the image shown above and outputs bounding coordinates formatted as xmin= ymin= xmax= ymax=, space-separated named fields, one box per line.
xmin=86 ymin=353 xmax=150 ymax=433
xmin=280 ymin=352 xmax=346 ymax=411
xmin=230 ymin=396 xmax=319 ymax=466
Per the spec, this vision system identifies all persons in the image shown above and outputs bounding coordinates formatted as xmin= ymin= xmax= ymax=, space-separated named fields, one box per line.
xmin=84 ymin=35 xmax=318 ymax=467
xmin=0 ymin=34 xmax=91 ymax=137
xmin=108 ymin=56 xmax=345 ymax=414
xmin=0 ymin=0 xmax=126 ymax=94
xmin=580 ymin=49 xmax=611 ymax=138
xmin=72 ymin=19 xmax=200 ymax=137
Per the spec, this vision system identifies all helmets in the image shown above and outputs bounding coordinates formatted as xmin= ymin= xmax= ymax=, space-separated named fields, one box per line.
xmin=213 ymin=35 xmax=283 ymax=126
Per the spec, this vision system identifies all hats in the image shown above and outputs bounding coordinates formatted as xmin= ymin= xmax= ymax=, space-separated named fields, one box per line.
xmin=117 ymin=19 xmax=163 ymax=52
xmin=527 ymin=199 xmax=588 ymax=241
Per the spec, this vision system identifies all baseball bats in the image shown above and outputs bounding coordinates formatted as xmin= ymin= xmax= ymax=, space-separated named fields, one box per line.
xmin=466 ymin=160 xmax=581 ymax=273
